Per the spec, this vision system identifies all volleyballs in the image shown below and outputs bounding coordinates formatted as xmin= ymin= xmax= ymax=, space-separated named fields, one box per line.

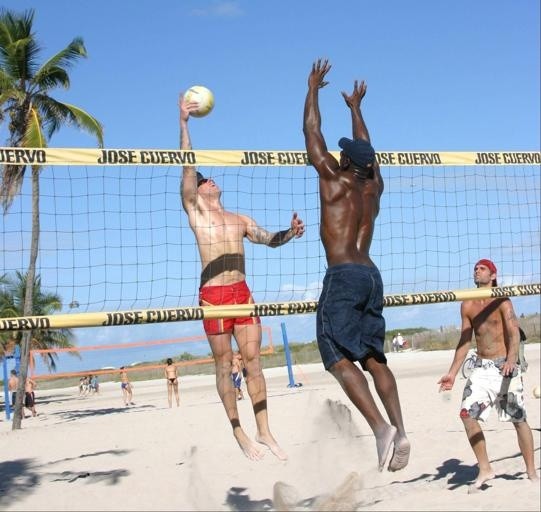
xmin=184 ymin=86 xmax=213 ymax=116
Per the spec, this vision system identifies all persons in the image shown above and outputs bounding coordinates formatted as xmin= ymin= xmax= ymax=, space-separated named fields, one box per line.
xmin=226 ymin=349 xmax=249 ymax=401
xmin=392 ymin=335 xmax=397 ymax=353
xmin=81 ymin=374 xmax=99 ymax=397
xmin=397 ymin=333 xmax=403 ymax=354
xmin=119 ymin=367 xmax=133 ymax=406
xmin=303 ymin=57 xmax=411 ymax=472
xmin=438 ymin=258 xmax=540 ymax=489
xmin=177 ymin=96 xmax=304 ymax=461
xmin=8 ymin=369 xmax=25 ymax=420
xmin=25 ymin=379 xmax=38 ymax=417
xmin=164 ymin=358 xmax=180 ymax=408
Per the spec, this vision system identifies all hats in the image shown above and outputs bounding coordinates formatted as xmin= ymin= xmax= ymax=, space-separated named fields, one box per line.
xmin=337 ymin=136 xmax=376 ymax=169
xmin=196 ymin=171 xmax=205 ymax=182
xmin=476 ymin=258 xmax=499 ymax=275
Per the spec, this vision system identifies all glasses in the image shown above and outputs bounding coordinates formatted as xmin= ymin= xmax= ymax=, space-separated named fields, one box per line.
xmin=198 ymin=178 xmax=210 ymax=186
